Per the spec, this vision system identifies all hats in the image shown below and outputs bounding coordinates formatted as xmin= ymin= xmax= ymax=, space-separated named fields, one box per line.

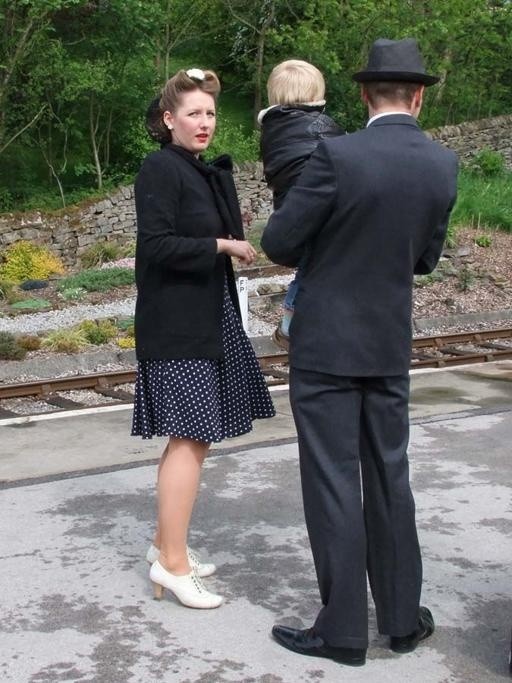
xmin=352 ymin=38 xmax=440 ymax=86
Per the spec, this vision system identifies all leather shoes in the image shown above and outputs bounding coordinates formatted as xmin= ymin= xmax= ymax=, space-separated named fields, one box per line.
xmin=390 ymin=607 xmax=435 ymax=653
xmin=272 ymin=625 xmax=366 ymax=667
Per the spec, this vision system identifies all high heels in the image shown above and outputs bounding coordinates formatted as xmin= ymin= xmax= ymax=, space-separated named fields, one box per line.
xmin=146 ymin=544 xmax=223 ymax=609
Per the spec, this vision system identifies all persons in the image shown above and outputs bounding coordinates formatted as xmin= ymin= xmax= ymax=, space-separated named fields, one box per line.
xmin=260 ymin=35 xmax=462 ymax=669
xmin=254 ymin=58 xmax=345 ymax=353
xmin=131 ymin=65 xmax=274 ymax=612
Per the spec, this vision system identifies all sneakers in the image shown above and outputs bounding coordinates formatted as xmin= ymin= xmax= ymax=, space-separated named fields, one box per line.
xmin=273 ymin=321 xmax=290 ymax=352
